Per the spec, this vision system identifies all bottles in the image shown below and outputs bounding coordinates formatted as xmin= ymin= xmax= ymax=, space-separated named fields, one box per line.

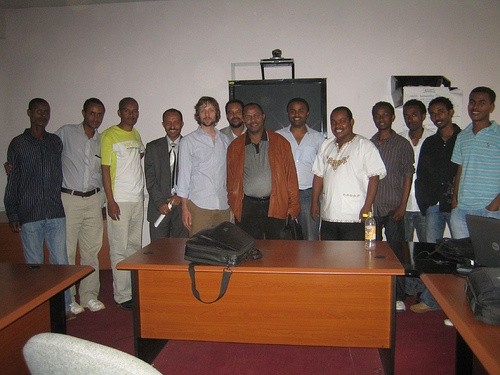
xmin=365 ymin=212 xmax=376 ymax=250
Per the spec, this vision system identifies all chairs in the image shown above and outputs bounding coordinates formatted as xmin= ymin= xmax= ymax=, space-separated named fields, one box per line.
xmin=23 ymin=332 xmax=163 ymax=375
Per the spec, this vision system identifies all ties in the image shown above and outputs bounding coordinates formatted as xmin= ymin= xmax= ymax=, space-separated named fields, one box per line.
xmin=169 ymin=143 xmax=176 ymax=189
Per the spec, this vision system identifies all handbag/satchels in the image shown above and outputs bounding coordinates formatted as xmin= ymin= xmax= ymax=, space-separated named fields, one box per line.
xmin=184 ymin=221 xmax=263 ymax=267
xmin=465 ymin=266 xmax=500 ymax=325
xmin=287 ymin=214 xmax=303 ymax=240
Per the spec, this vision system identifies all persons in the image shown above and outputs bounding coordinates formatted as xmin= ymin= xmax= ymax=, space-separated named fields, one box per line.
xmin=100 ymin=98 xmax=146 ymax=310
xmin=275 ymin=97 xmax=325 ymax=240
xmin=4 ymin=98 xmax=77 ymax=321
xmin=410 ymin=97 xmax=461 ymax=313
xmin=176 ymin=96 xmax=232 ymax=237
xmin=396 ymin=99 xmax=436 ymax=298
xmin=226 ymin=103 xmax=299 ymax=239
xmin=4 ymin=98 xmax=107 ymax=314
xmin=369 ymin=102 xmax=415 ymax=312
xmin=218 ymin=100 xmax=247 ymax=141
xmin=144 ymin=109 xmax=189 ymax=241
xmin=310 ymin=106 xmax=387 ymax=241
xmin=445 ymin=86 xmax=500 ymax=326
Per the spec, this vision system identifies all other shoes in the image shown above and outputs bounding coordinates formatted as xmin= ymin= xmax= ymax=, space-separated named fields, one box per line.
xmin=120 ymin=300 xmax=132 ymax=309
xmin=83 ymin=299 xmax=105 ymax=311
xmin=410 ymin=301 xmax=441 ymax=313
xmin=66 ymin=311 xmax=76 ymax=320
xmin=396 ymin=301 xmax=406 ymax=312
xmin=69 ymin=303 xmax=85 ymax=314
xmin=444 ymin=320 xmax=453 ymax=326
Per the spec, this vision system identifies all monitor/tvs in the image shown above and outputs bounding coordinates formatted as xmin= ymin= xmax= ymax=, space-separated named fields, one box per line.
xmin=229 ymin=77 xmax=327 ymax=138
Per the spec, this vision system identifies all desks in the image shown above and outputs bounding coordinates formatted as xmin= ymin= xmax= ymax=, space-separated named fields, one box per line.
xmin=420 ymin=272 xmax=500 ymax=375
xmin=116 ymin=237 xmax=406 ymax=375
xmin=0 ymin=261 xmax=96 ymax=335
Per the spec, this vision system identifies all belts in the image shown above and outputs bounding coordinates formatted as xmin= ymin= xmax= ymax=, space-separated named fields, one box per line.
xmin=244 ymin=194 xmax=270 ymax=201
xmin=60 ymin=187 xmax=100 ymax=198
